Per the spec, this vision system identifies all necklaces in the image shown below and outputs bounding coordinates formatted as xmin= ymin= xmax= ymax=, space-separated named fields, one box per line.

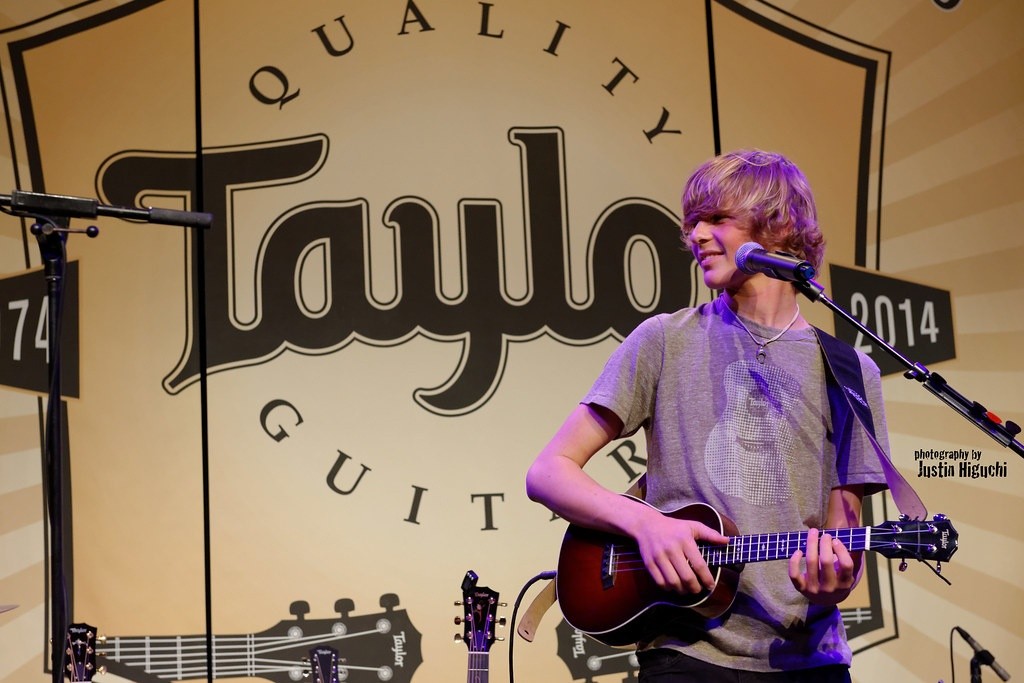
xmin=720 ymin=295 xmax=799 ymax=364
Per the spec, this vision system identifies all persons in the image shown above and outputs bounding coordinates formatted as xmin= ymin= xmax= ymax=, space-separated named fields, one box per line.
xmin=525 ymin=147 xmax=891 ymax=683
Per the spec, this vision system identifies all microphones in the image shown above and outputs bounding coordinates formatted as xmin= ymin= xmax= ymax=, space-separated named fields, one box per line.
xmin=955 ymin=627 xmax=1011 ymax=682
xmin=734 ymin=241 xmax=816 ymax=284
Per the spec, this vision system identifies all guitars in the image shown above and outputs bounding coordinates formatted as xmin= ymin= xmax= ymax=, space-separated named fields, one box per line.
xmin=300 ymin=646 xmax=342 ymax=682
xmin=557 ymin=492 xmax=959 ymax=647
xmin=63 ymin=621 xmax=108 ymax=683
xmin=453 ymin=570 xmax=508 ymax=683
xmin=95 ymin=593 xmax=425 ymax=682
